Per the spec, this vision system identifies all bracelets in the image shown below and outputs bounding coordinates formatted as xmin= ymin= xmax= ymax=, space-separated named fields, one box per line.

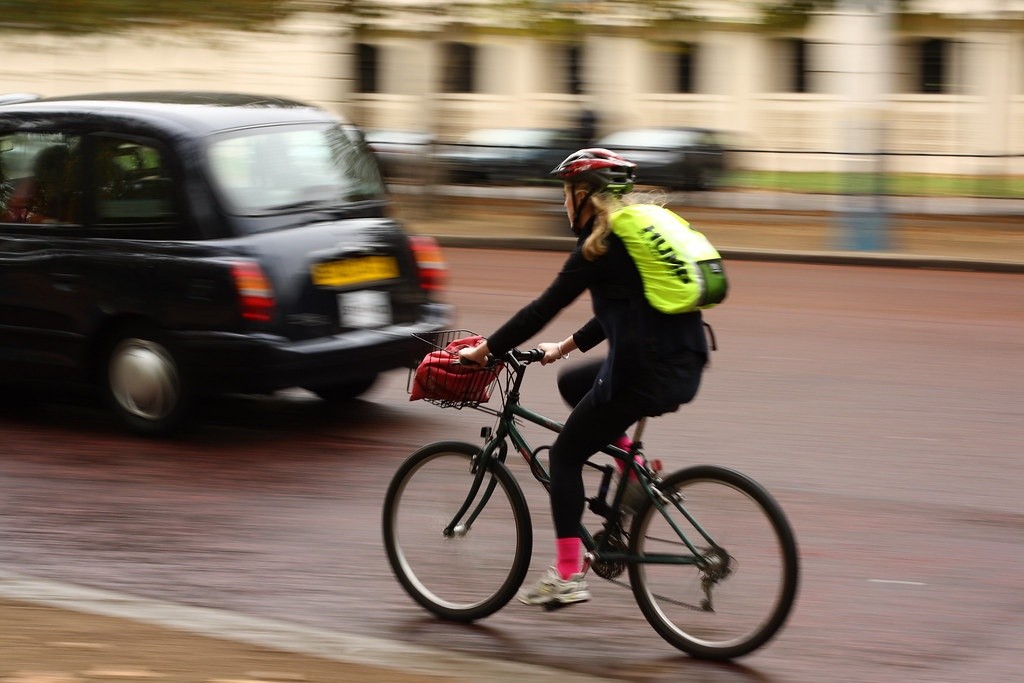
xmin=557 ymin=343 xmax=569 ymax=359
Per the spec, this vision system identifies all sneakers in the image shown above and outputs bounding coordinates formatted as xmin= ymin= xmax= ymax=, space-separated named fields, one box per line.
xmin=517 ymin=567 xmax=591 ymax=606
xmin=616 ymin=470 xmax=659 ymax=514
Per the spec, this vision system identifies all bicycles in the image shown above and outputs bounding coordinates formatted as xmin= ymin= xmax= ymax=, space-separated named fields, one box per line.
xmin=379 ymin=328 xmax=801 ymax=661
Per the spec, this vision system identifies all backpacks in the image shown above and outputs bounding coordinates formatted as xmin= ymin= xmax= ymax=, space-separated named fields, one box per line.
xmin=608 ymin=203 xmax=729 ymax=315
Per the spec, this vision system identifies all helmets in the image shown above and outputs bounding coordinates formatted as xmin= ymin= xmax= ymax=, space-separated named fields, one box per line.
xmin=547 ymin=148 xmax=637 ymax=192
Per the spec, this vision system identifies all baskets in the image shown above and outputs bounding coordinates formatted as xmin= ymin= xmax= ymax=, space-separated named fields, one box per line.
xmin=407 ymin=329 xmax=509 ymax=410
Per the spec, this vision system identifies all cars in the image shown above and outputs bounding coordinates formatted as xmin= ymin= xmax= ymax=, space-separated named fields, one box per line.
xmin=589 ymin=125 xmax=729 ymax=190
xmin=0 ymin=90 xmax=453 ymax=438
xmin=440 ymin=117 xmax=599 ymax=184
xmin=355 ymin=123 xmax=442 ymax=183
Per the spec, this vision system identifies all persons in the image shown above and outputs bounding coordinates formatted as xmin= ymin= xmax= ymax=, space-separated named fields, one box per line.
xmin=462 ymin=145 xmax=705 ymax=606
xmin=8 ymin=147 xmax=78 ymax=229
